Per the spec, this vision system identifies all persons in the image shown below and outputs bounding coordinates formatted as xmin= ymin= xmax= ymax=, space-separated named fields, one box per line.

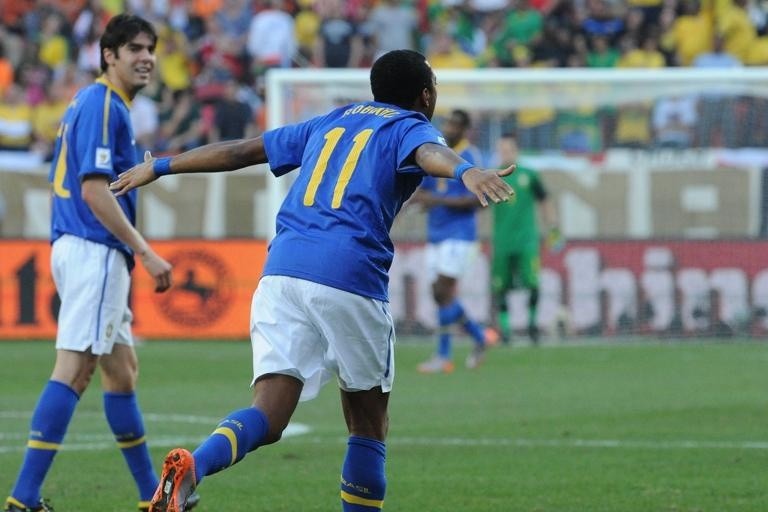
xmin=111 ymin=48 xmax=520 ymax=511
xmin=5 ymin=15 xmax=169 ymax=512
xmin=490 ymin=133 xmax=553 ymax=345
xmin=405 ymin=110 xmax=499 ymax=373
xmin=0 ymin=1 xmax=768 ymax=162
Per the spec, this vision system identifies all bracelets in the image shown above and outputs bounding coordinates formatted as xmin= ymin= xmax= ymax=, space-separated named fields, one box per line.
xmin=152 ymin=158 xmax=173 ymax=175
xmin=455 ymin=162 xmax=475 ymax=180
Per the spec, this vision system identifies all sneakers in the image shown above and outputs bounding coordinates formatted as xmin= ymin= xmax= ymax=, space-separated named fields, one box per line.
xmin=3 ymin=495 xmax=54 ymax=511
xmin=138 ymin=448 xmax=199 ymax=512
xmin=464 ymin=328 xmax=498 ymax=371
xmin=416 ymin=355 xmax=454 ymax=374
